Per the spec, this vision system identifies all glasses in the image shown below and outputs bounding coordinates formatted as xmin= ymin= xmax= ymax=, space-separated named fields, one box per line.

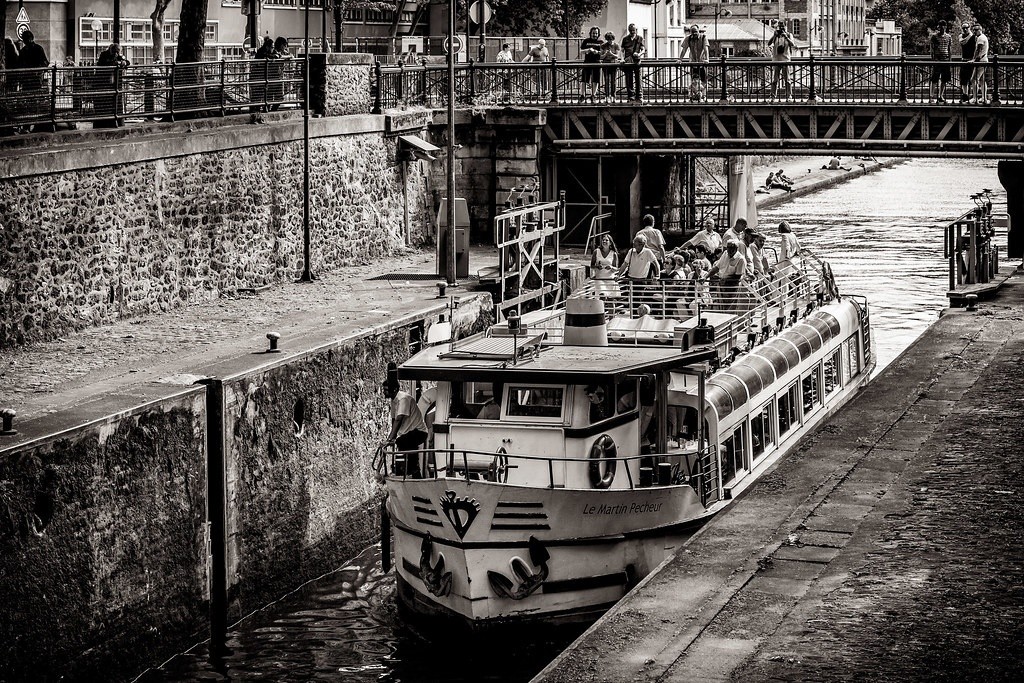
xmin=962 ymin=27 xmax=969 ymax=29
xmin=973 ymin=29 xmax=980 ymax=32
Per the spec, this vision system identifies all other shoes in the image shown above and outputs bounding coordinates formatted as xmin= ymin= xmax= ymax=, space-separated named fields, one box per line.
xmin=960 ymin=95 xmax=967 ymax=103
xmin=979 ymin=98 xmax=987 ymax=103
xmin=579 ymin=95 xmax=585 ymax=104
xmin=937 ymin=97 xmax=947 ymax=103
xmin=929 ymin=96 xmax=936 ymax=104
xmin=966 ymin=99 xmax=979 ymax=104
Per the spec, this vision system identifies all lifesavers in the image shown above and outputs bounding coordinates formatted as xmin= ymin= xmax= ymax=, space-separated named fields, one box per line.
xmin=590 ymin=435 xmax=617 ymax=489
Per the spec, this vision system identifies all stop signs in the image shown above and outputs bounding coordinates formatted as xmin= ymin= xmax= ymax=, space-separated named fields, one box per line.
xmin=444 ymin=36 xmax=462 ymax=55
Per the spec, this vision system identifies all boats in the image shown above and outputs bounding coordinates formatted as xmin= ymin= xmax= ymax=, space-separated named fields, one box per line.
xmin=377 ymin=243 xmax=876 ymax=630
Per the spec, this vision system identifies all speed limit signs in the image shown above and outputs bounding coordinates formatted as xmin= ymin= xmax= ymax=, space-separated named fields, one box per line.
xmin=15 ymin=23 xmax=32 ymax=41
xmin=242 ymin=36 xmax=261 ymax=55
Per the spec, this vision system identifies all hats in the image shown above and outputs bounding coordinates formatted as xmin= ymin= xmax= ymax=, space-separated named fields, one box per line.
xmin=938 ymin=20 xmax=948 ymax=27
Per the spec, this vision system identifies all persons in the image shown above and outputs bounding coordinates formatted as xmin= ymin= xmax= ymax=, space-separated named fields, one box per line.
xmin=589 ymin=214 xmax=799 ymax=322
xmin=576 ymin=25 xmax=605 ymax=104
xmin=90 ymin=42 xmax=129 ymax=115
xmin=518 ymin=38 xmax=549 ymax=97
xmin=382 ymin=379 xmax=428 ymax=478
xmin=675 ymin=24 xmax=709 ymax=102
xmin=928 ymin=19 xmax=952 ymax=103
xmin=620 ymin=22 xmax=645 ymax=101
xmin=767 ymin=19 xmax=794 ymax=103
xmin=495 ymin=42 xmax=518 ymax=92
xmin=965 ymin=23 xmax=989 ymax=103
xmin=18 ymin=29 xmax=48 ymax=134
xmin=599 ymin=30 xmax=620 ymax=103
xmin=821 ymin=154 xmax=852 ymax=171
xmin=958 ymin=20 xmax=977 ymax=100
xmin=754 ymin=168 xmax=796 ymax=194
xmin=4 ymin=36 xmax=19 ymax=92
xmin=249 ymin=35 xmax=294 ymax=112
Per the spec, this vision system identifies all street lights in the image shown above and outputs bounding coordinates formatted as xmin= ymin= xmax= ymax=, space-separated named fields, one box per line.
xmin=808 ymin=22 xmax=824 ymax=55
xmin=713 ymin=3 xmax=733 ymax=87
xmin=90 ymin=19 xmax=102 ymax=74
xmin=761 ymin=14 xmax=778 ymax=55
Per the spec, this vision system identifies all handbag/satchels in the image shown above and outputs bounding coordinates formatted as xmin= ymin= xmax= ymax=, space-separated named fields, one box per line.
xmin=620 ymin=56 xmax=633 ymax=73
